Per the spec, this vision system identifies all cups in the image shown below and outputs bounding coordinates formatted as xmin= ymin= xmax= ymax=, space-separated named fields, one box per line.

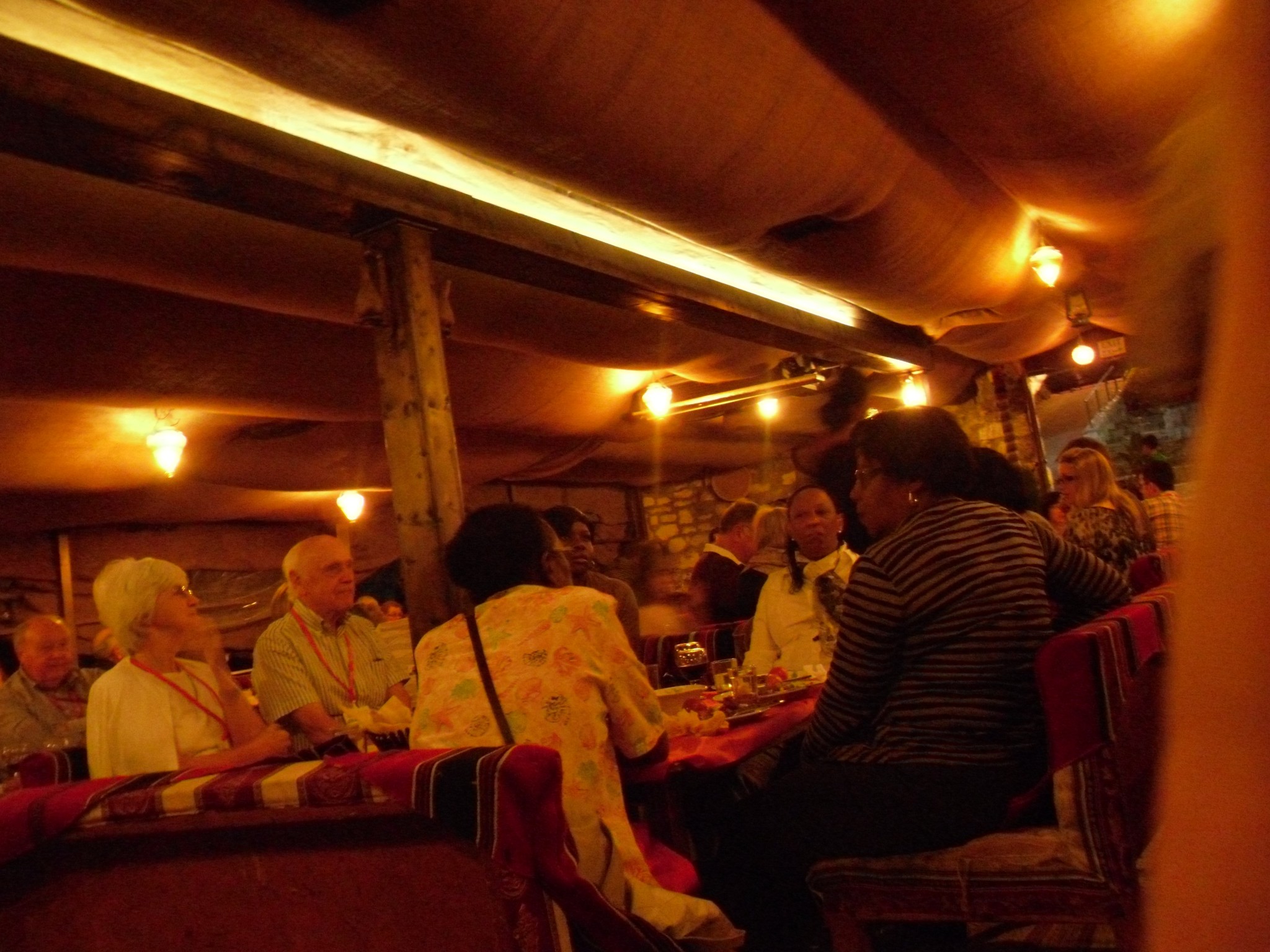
xmin=728 ymin=664 xmax=757 ymax=703
xmin=3 ymin=736 xmax=69 ymax=779
xmin=674 ymin=641 xmax=709 ymax=685
xmin=645 ymin=664 xmax=660 ymax=689
xmin=711 ymin=658 xmax=739 ymax=692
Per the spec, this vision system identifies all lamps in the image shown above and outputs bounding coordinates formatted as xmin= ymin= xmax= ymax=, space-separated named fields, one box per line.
xmin=1028 ymin=233 xmax=1063 ymax=287
xmin=642 ymin=370 xmax=673 ymax=420
xmin=146 ymin=408 xmax=187 ymax=479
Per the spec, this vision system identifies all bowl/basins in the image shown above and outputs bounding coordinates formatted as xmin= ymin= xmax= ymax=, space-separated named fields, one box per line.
xmin=653 ymin=684 xmax=708 ymax=715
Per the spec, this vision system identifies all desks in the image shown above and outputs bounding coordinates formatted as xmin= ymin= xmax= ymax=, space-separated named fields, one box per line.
xmin=621 ymin=681 xmax=822 ymax=952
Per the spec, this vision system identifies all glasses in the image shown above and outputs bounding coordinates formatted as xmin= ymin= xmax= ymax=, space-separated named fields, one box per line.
xmin=1054 ymin=475 xmax=1079 ymax=486
xmin=153 ymin=586 xmax=187 ymax=607
xmin=854 ymin=466 xmax=886 ymax=490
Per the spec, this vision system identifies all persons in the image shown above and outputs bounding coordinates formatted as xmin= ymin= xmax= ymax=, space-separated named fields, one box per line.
xmin=271 ymin=583 xmax=293 ymax=619
xmin=86 ymin=557 xmax=294 ymax=780
xmin=718 ymin=406 xmax=1133 ymax=951
xmin=251 ymin=534 xmax=415 ymax=751
xmin=410 ymin=505 xmax=745 ymax=952
xmin=744 ymin=484 xmax=860 ymax=685
xmin=0 ymin=615 xmax=126 ymax=766
xmin=544 ymin=507 xmax=640 ymax=660
xmin=1042 ymin=434 xmax=1178 ymax=577
xmin=358 ymin=597 xmax=414 ymax=675
xmin=688 ymin=499 xmax=788 ymax=658
xmin=977 ymin=446 xmax=1093 ymax=624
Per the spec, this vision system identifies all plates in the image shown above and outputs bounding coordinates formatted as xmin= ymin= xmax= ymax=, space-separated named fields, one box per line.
xmin=712 ymin=681 xmax=814 ymax=702
xmin=724 ymin=706 xmax=771 ymax=720
xmin=730 ymin=670 xmax=812 ymax=688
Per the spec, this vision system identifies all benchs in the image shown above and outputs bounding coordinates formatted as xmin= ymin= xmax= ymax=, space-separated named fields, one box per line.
xmin=0 ymin=551 xmax=1171 ymax=952
